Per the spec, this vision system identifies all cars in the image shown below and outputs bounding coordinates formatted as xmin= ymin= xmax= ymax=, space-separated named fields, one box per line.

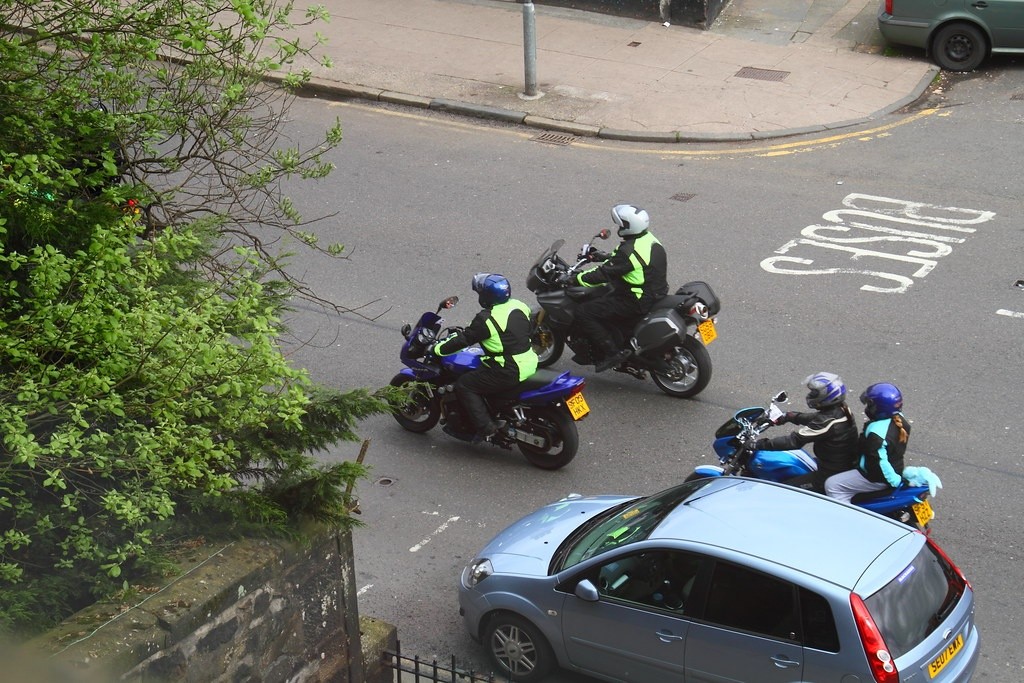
xmin=875 ymin=1 xmax=1024 ymax=72
xmin=459 ymin=474 xmax=983 ymax=683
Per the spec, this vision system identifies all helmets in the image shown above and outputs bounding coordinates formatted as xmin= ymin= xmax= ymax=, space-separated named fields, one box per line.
xmin=471 ymin=271 xmax=511 ymax=308
xmin=806 ymin=372 xmax=846 ymax=409
xmin=860 ymin=382 xmax=903 ymax=421
xmin=611 ymin=204 xmax=650 ymax=237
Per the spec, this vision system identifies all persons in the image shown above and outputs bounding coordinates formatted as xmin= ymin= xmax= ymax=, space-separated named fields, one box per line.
xmin=556 ymin=205 xmax=669 ymax=373
xmin=424 ymin=272 xmax=538 ymax=445
xmin=824 ymin=383 xmax=911 ymax=504
xmin=444 ymin=300 xmax=453 ymax=308
xmin=741 ymin=372 xmax=858 ymax=494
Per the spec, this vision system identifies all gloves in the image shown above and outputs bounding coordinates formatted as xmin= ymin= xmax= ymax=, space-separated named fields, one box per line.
xmin=581 ymin=245 xmax=611 ymax=262
xmin=422 ymin=344 xmax=435 ymax=354
xmin=554 ymin=273 xmax=578 ymax=287
xmin=743 ymin=439 xmax=757 ymax=451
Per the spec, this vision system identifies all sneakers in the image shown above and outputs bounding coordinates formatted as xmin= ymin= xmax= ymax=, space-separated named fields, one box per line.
xmin=471 ymin=421 xmax=496 ymax=446
xmin=595 ymin=352 xmax=627 ymax=373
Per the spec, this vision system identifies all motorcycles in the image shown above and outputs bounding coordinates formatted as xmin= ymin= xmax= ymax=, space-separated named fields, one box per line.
xmin=514 ymin=229 xmax=724 ymax=397
xmin=685 ymin=391 xmax=938 ymax=536
xmin=388 ymin=295 xmax=589 ymax=471
